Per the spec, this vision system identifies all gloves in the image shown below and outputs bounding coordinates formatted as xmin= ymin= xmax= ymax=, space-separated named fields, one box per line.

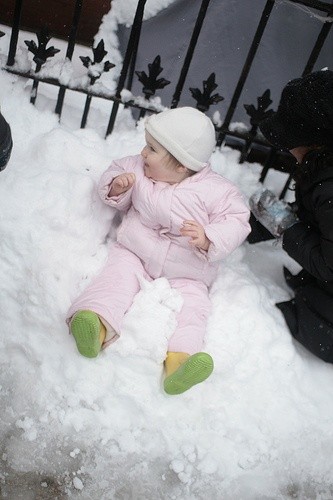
xmin=248 ymin=189 xmax=299 ymax=238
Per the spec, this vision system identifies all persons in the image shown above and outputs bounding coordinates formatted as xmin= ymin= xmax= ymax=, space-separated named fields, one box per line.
xmin=249 ymin=71 xmax=333 ymax=366
xmin=65 ymin=106 xmax=251 ymax=396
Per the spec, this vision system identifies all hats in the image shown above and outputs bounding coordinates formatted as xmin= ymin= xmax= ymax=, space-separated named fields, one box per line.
xmin=144 ymin=106 xmax=216 ymax=172
xmin=272 ymin=71 xmax=333 ymax=151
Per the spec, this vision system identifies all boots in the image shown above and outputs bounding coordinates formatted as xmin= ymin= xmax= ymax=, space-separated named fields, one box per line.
xmin=71 ymin=309 xmax=107 ymax=358
xmin=163 ymin=351 xmax=213 ymax=395
